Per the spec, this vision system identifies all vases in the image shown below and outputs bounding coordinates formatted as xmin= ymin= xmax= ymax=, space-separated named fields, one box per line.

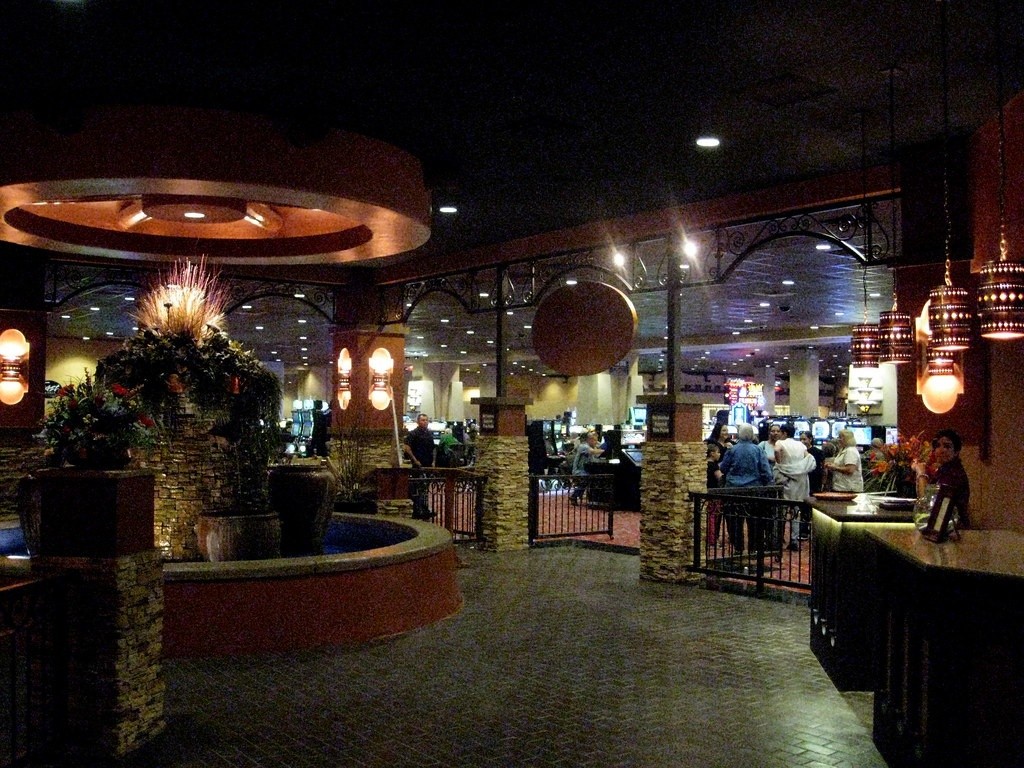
xmin=37 ymin=469 xmax=157 ymax=557
xmin=268 ymin=465 xmax=337 ymax=558
xmin=895 ymin=480 xmax=917 ymax=499
xmin=197 ymin=508 xmax=282 ymax=562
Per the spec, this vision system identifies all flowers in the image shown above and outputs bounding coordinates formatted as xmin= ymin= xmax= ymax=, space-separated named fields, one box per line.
xmin=859 ymin=429 xmax=933 ymax=497
xmin=20 ymin=368 xmax=170 ymax=468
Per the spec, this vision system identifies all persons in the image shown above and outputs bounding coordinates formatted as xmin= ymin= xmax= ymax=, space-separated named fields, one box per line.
xmin=282 ymin=421 xmax=292 ymax=433
xmin=911 ymin=429 xmax=970 ymax=530
xmin=435 ymin=419 xmax=478 ymax=467
xmin=704 ymin=422 xmax=884 ymax=553
xmin=546 ymin=431 xmax=607 ymax=506
xmin=404 ymin=414 xmax=436 ymax=520
xmin=310 ymin=400 xmax=327 ymax=457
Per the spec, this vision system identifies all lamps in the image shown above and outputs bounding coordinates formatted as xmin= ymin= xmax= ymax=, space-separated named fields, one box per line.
xmin=848 ymin=0 xmax=1024 ymax=415
xmin=337 ymin=347 xmax=352 ymax=411
xmin=0 ymin=328 xmax=30 ymax=405
xmin=368 ymin=347 xmax=395 ymax=411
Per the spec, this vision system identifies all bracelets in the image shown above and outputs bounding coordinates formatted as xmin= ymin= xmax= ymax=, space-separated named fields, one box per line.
xmin=917 ymin=474 xmax=929 ymax=480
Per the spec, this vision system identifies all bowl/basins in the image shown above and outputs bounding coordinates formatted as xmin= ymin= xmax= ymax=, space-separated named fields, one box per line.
xmin=195 ymin=510 xmax=281 ymax=561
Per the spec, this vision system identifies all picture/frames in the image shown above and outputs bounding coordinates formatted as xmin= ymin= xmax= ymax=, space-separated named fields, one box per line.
xmin=922 ymin=484 xmax=963 ymax=544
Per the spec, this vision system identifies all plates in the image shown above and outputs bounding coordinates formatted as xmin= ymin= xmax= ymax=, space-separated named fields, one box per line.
xmin=813 ymin=491 xmax=858 ymax=500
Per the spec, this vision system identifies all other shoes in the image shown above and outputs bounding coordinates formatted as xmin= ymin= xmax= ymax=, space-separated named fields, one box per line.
xmin=733 ymin=551 xmax=744 ymax=557
xmin=749 ymin=552 xmax=757 ymax=559
xmin=569 ymin=497 xmax=579 ymax=506
xmin=798 ymin=534 xmax=809 ymax=539
xmin=710 ymin=543 xmax=726 ymax=549
xmin=786 ymin=542 xmax=799 ymax=551
xmin=764 ymin=546 xmax=780 ymax=554
xmin=422 ymin=512 xmax=436 ymax=520
xmin=412 ymin=512 xmax=422 ymax=519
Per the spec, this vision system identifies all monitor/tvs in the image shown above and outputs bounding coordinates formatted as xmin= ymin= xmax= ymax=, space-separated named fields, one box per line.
xmin=541 ymin=420 xmax=565 ymax=456
xmin=771 ymin=419 xmax=899 ymax=448
xmin=290 ymin=411 xmax=315 ymax=437
xmin=625 ymin=451 xmax=642 ymax=462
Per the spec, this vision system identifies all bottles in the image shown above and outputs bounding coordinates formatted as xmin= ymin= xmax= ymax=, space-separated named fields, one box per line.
xmin=267 ymin=464 xmax=334 ymax=552
